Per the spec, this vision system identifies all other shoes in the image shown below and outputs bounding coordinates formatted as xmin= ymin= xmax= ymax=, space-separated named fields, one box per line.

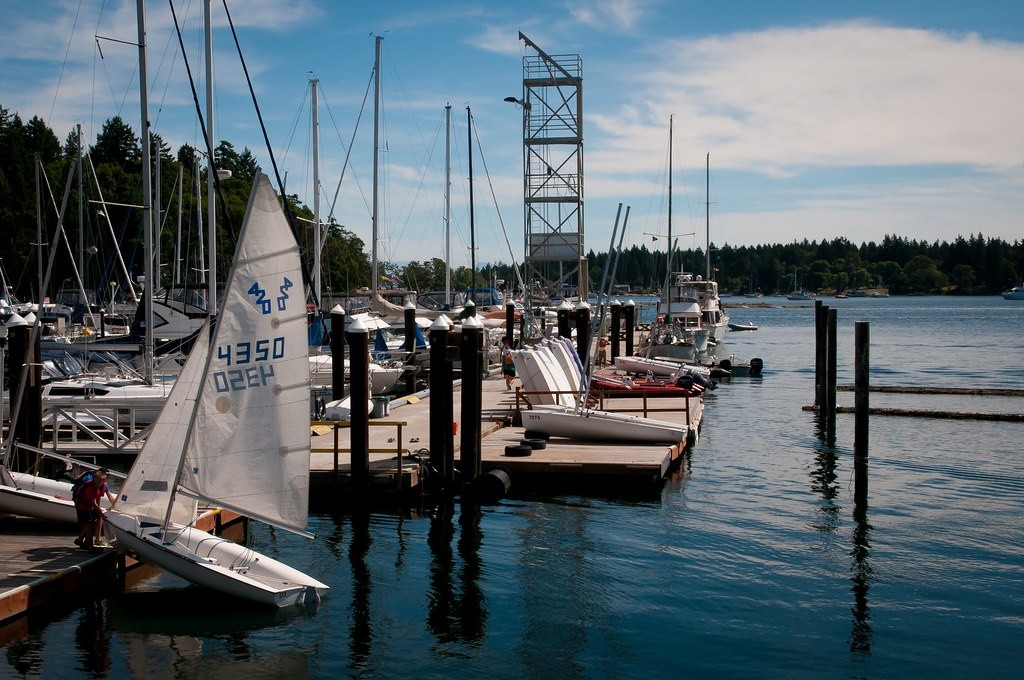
xmin=74 ymin=538 xmax=85 ymax=547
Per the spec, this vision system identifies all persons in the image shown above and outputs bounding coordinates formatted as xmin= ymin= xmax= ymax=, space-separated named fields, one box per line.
xmin=598 ymin=335 xmax=610 ymax=370
xmin=499 ymin=337 xmax=516 ymax=390
xmin=71 ymin=467 xmax=114 ymax=550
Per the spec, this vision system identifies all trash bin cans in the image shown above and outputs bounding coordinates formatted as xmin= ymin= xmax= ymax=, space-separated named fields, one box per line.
xmin=369 ymin=396 xmax=389 ymax=418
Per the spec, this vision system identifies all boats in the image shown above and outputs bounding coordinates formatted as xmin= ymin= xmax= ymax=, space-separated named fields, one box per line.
xmin=744 ymin=287 xmax=764 ymax=298
xmin=786 ymin=272 xmax=817 ymax=300
xmin=835 ymin=295 xmax=849 ymax=299
xmin=1002 ymin=286 xmax=1024 ymax=300
xmin=729 ymin=323 xmax=759 ymax=330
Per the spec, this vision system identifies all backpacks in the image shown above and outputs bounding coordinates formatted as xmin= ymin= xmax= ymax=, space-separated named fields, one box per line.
xmin=504 ymin=349 xmax=513 ymax=365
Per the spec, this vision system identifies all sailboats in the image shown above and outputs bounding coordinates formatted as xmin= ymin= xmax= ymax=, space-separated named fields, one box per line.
xmin=0 ymin=1 xmax=722 ymax=606
